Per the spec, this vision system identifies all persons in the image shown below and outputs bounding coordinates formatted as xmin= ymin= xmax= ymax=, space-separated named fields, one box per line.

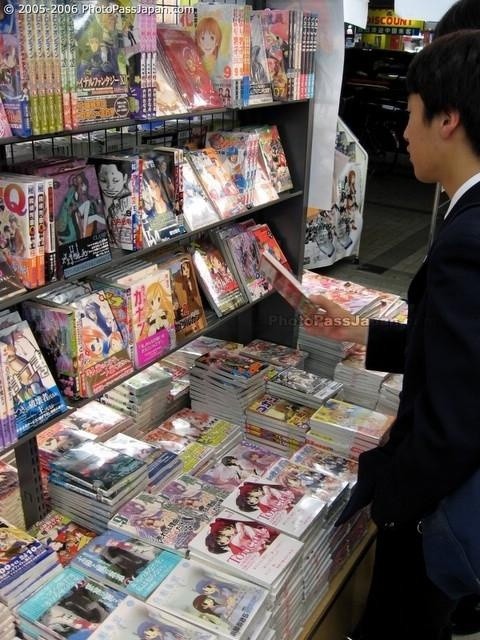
xmin=303 ymin=28 xmax=480 ymax=640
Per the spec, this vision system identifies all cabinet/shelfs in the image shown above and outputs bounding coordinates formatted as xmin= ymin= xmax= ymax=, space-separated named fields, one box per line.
xmin=2 ymin=0 xmax=418 ymax=640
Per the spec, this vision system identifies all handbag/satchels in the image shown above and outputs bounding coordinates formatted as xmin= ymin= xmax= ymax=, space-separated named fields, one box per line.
xmin=417 ymin=474 xmax=480 ymax=600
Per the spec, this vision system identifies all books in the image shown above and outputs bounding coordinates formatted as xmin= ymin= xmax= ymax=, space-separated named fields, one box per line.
xmin=301 ymin=271 xmax=414 ymax=413
xmin=247 ymin=369 xmax=399 ymax=459
